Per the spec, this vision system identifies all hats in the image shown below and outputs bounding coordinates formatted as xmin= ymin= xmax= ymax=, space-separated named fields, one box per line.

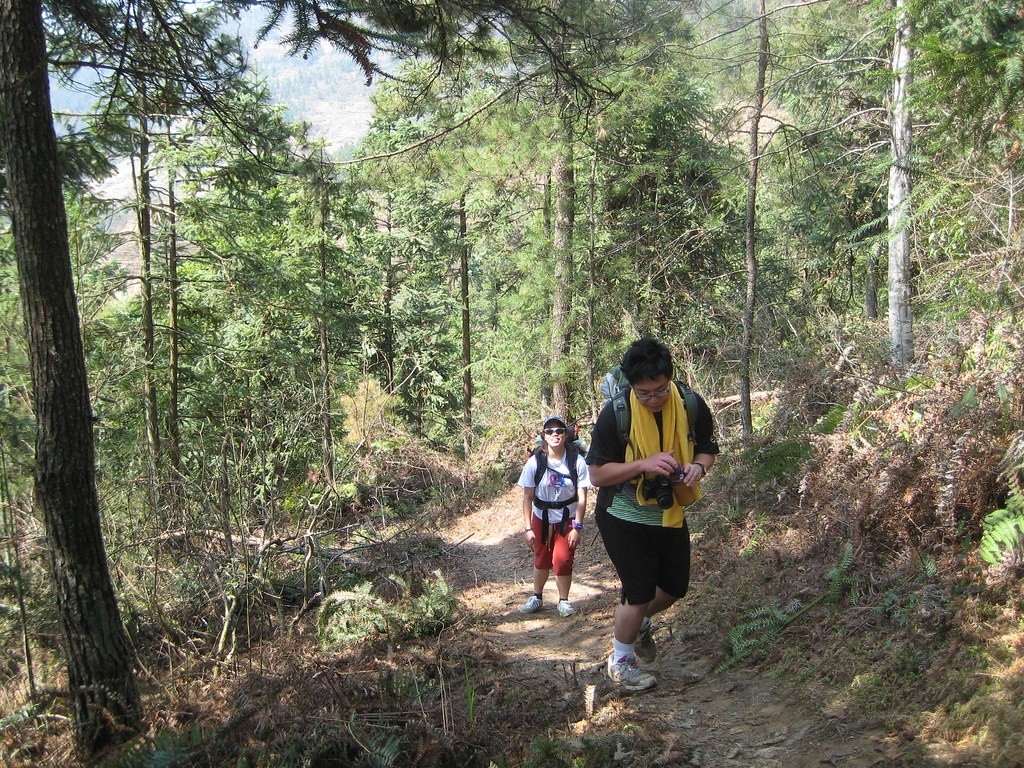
xmin=542 ymin=416 xmax=569 ymax=430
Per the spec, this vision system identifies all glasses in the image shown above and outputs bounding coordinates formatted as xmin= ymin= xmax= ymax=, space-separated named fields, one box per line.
xmin=634 ymin=379 xmax=670 ymax=400
xmin=544 ymin=428 xmax=568 ymax=435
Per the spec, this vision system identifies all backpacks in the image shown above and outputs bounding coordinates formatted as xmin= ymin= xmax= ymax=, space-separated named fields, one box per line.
xmin=600 ymin=365 xmax=698 ymax=447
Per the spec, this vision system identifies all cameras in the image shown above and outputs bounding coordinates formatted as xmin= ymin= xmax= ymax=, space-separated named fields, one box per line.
xmin=642 ymin=474 xmax=674 ymax=509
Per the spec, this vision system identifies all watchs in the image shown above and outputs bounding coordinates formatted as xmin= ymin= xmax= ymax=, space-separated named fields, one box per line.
xmin=694 ymin=462 xmax=707 ymax=477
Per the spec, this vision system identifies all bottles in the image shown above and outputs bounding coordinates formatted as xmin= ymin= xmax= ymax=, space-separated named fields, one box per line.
xmin=667 ymin=460 xmax=696 ymax=508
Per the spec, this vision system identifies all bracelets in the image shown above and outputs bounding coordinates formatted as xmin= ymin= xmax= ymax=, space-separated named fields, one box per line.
xmin=524 ymin=528 xmax=533 ymax=532
xmin=571 ymin=520 xmax=583 ymax=530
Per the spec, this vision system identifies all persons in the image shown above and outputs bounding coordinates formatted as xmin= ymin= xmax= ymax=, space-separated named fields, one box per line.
xmin=584 ymin=337 xmax=719 ymax=690
xmin=514 ymin=417 xmax=592 ymax=616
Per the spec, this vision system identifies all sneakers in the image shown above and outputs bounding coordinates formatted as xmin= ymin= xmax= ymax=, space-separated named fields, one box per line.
xmin=634 ymin=618 xmax=656 ymax=663
xmin=518 ymin=595 xmax=544 ymax=613
xmin=556 ymin=600 xmax=576 ymax=617
xmin=608 ymin=651 xmax=656 ymax=690
